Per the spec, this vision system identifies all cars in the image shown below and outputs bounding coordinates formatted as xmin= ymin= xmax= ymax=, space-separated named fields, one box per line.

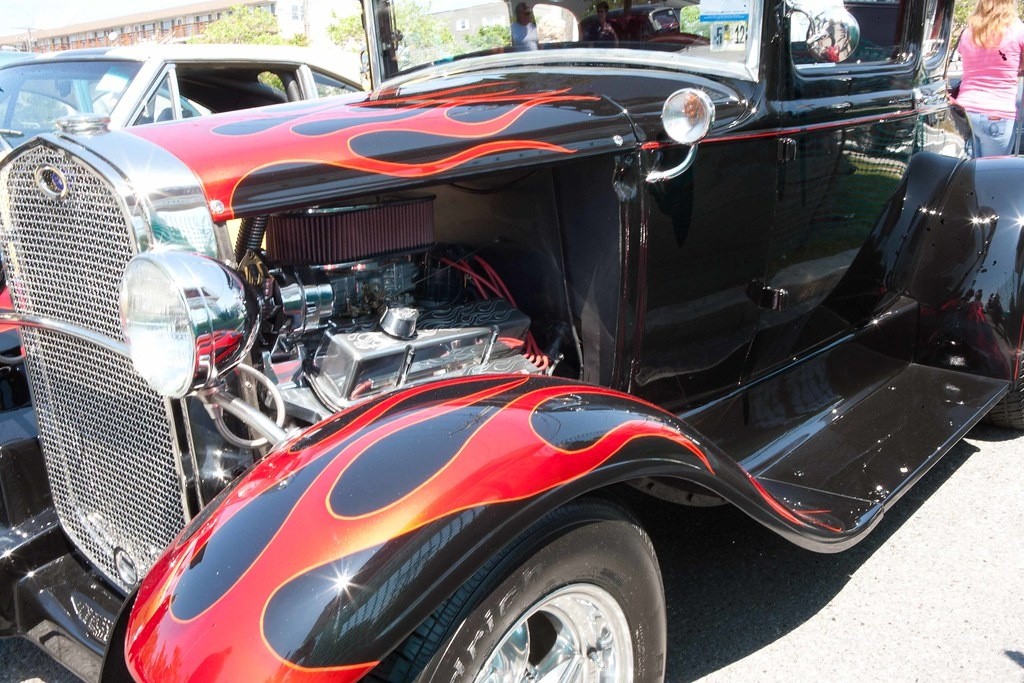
xmin=0 ymin=0 xmax=1023 ymax=683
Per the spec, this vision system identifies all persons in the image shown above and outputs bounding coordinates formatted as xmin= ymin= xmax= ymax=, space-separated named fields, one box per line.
xmin=511 ymin=2 xmax=538 ymax=45
xmin=955 ymin=0 xmax=1024 ymax=160
xmin=589 ymin=1 xmax=619 ymax=41
xmin=814 ymin=18 xmax=852 ymax=62
